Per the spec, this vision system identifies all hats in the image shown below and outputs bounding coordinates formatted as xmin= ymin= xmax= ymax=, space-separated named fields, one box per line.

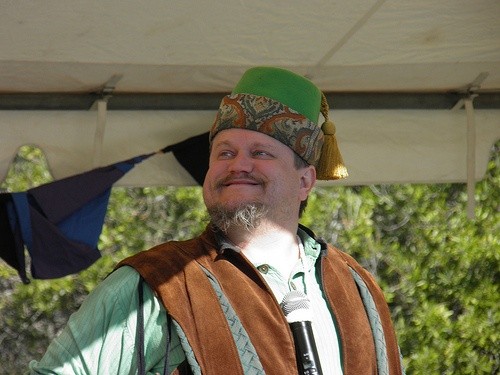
xmin=209 ymin=64 xmax=349 ymax=180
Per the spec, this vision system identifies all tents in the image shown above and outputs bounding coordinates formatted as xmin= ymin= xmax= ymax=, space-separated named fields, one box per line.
xmin=1 ymin=0 xmax=499 ymax=187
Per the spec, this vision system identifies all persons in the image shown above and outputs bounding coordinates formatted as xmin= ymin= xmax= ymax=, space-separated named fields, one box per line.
xmin=23 ymin=65 xmax=408 ymax=375
